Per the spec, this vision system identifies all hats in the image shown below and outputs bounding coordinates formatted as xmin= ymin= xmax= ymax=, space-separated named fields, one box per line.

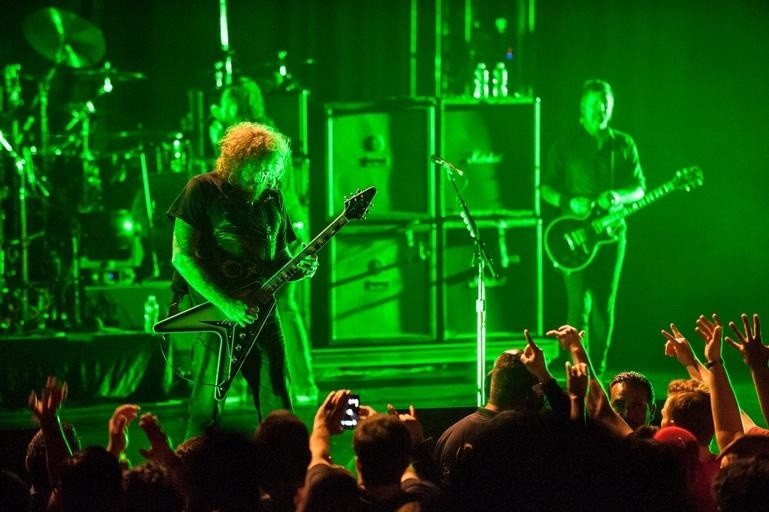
xmin=654 ymin=425 xmax=700 ymax=482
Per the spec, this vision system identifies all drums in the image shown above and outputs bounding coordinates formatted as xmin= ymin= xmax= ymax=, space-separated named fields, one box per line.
xmin=79 ymin=209 xmax=134 ymax=261
xmin=95 ymin=78 xmax=183 ymax=149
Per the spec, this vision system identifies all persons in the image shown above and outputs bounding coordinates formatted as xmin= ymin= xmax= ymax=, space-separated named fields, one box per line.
xmin=0 ymin=313 xmax=768 ymax=512
xmin=537 ymin=76 xmax=646 ymax=379
xmin=207 ymin=76 xmax=322 ymax=405
xmin=164 ymin=114 xmax=319 ymax=440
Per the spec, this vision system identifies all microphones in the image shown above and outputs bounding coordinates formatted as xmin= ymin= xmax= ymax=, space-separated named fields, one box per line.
xmin=432 ymin=154 xmax=462 ymax=176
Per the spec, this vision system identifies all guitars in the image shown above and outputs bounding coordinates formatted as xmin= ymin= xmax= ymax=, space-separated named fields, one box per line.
xmin=152 ymin=186 xmax=375 ymax=403
xmin=543 ymin=166 xmax=703 ymax=274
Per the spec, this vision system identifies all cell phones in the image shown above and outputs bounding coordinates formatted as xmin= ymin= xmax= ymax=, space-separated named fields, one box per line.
xmin=338 ymin=393 xmax=360 ymax=430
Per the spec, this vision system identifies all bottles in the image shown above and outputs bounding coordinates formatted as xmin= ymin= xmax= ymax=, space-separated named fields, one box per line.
xmin=472 ymin=63 xmax=489 ymax=98
xmin=143 ymin=294 xmax=160 ymax=333
xmin=493 ymin=61 xmax=508 ymax=100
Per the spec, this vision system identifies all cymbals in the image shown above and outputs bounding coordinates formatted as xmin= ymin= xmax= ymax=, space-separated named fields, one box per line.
xmin=23 ymin=7 xmax=106 ymax=69
